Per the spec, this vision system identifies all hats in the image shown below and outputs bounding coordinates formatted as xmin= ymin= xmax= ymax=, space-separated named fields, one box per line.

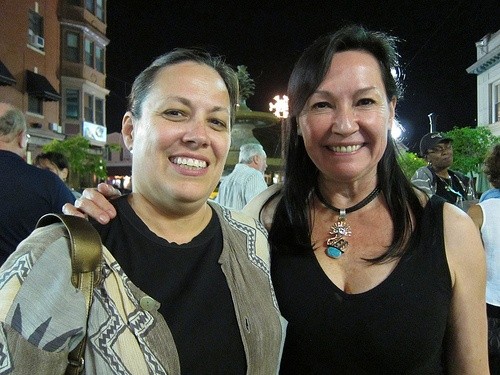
xmin=420 ymin=133 xmax=454 ymax=156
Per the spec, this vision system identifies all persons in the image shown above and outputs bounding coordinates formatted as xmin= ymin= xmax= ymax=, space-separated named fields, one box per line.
xmin=0 ymin=102 xmax=77 ymax=267
xmin=0 ymin=48 xmax=282 ymax=375
xmin=411 ymin=132 xmax=480 ymax=214
xmin=466 ymin=142 xmax=500 ymax=375
xmin=31 ymin=151 xmax=82 ymax=201
xmin=214 ymin=143 xmax=268 ymax=210
xmin=62 ymin=23 xmax=491 ymax=375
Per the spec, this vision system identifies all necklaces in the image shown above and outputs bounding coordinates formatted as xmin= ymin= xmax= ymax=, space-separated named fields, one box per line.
xmin=315 ymin=180 xmax=383 ymax=259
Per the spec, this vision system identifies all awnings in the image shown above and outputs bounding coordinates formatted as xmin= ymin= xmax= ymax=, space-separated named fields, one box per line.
xmin=0 ymin=60 xmax=18 ymax=86
xmin=27 ymin=71 xmax=62 ymax=102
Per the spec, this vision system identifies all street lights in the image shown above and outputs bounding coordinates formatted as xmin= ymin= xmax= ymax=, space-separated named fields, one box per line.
xmin=268 ymin=95 xmax=289 ymax=162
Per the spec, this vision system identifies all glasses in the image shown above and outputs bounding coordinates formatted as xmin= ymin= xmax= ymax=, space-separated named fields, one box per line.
xmin=429 ymin=145 xmax=452 ymax=153
xmin=19 ymin=129 xmax=31 ymax=139
